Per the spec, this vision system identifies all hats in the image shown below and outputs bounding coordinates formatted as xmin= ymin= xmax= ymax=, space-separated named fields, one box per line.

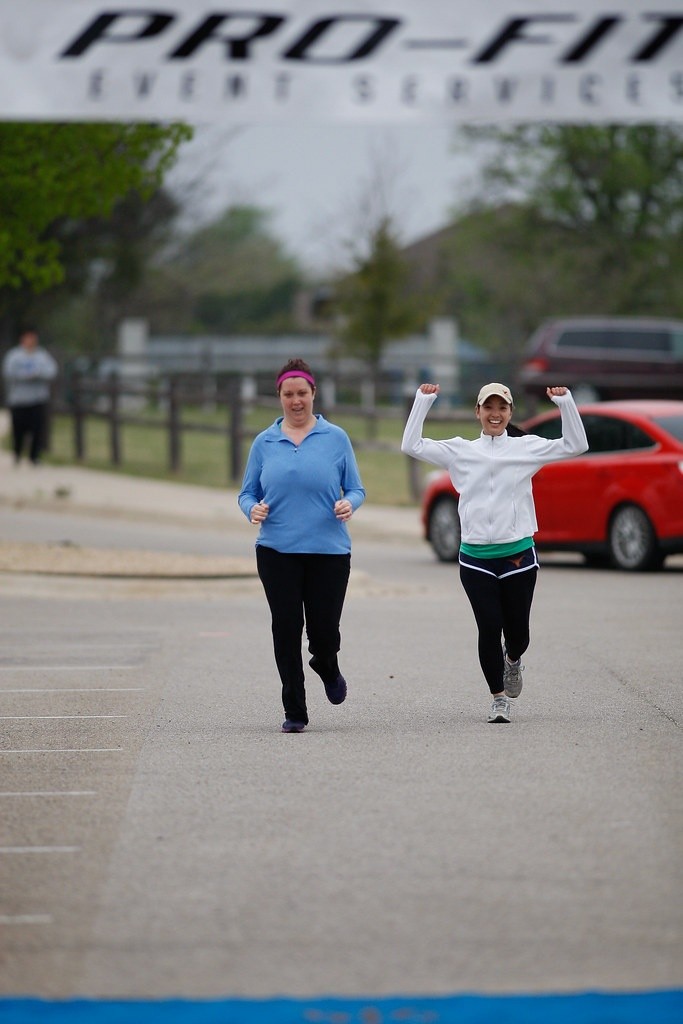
xmin=477 ymin=382 xmax=514 ymax=408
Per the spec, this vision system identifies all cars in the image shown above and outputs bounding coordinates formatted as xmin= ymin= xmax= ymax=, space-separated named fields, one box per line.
xmin=420 ymin=401 xmax=683 ymax=575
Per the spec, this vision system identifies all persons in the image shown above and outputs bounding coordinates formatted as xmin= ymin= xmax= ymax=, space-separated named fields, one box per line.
xmin=2 ymin=330 xmax=60 ymax=462
xmin=236 ymin=357 xmax=366 ymax=732
xmin=400 ymin=381 xmax=591 ymax=722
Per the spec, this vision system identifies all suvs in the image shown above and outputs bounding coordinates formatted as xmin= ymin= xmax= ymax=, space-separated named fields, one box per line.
xmin=515 ymin=319 xmax=683 ymax=400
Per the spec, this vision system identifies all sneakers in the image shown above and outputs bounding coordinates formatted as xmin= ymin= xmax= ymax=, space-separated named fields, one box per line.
xmin=282 ymin=720 xmax=306 ymax=733
xmin=487 ymin=695 xmax=510 ymax=723
xmin=502 ymin=641 xmax=522 ymax=699
xmin=308 ymin=656 xmax=347 ymax=705
